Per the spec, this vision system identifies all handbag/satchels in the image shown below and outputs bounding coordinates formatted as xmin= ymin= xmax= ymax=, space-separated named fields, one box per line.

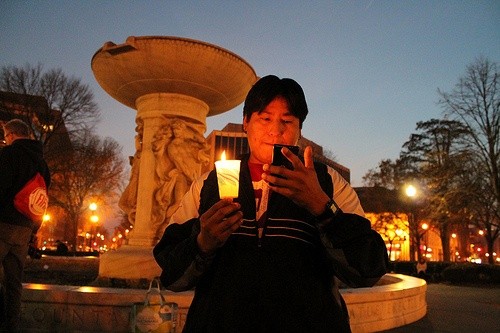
xmin=317 ymin=201 xmax=392 ymax=288
xmin=132 ymin=279 xmax=177 ymax=333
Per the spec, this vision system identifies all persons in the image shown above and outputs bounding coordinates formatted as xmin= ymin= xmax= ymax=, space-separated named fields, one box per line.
xmin=153 ymin=75 xmax=388 ymax=333
xmin=0 ymin=119 xmax=68 ymax=333
xmin=158 ymin=121 xmax=203 ymax=233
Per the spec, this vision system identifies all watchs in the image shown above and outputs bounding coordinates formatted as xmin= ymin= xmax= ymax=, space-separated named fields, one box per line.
xmin=316 ymin=200 xmax=340 ymax=224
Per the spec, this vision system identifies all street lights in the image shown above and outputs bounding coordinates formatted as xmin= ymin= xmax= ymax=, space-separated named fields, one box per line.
xmin=402 ymin=175 xmax=421 ymax=262
xmin=88 ymin=201 xmax=101 ymax=252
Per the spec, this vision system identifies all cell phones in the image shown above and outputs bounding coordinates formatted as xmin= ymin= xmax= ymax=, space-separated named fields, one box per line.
xmin=269 ymin=144 xmax=299 ymax=187
